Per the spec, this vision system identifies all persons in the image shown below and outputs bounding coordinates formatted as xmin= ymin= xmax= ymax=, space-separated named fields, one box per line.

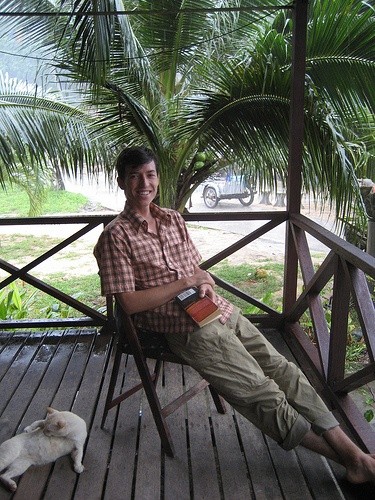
xmin=93 ymin=144 xmax=373 ymax=486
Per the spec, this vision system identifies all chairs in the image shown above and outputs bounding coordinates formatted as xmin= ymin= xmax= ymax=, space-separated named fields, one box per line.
xmin=99 ymin=273 xmax=226 ymax=458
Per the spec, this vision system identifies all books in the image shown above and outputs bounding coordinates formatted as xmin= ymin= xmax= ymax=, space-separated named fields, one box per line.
xmin=173 ymin=286 xmax=223 ymax=328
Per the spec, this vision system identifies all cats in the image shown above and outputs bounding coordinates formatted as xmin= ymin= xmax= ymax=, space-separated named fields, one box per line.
xmin=0 ymin=407 xmax=87 ymax=492
